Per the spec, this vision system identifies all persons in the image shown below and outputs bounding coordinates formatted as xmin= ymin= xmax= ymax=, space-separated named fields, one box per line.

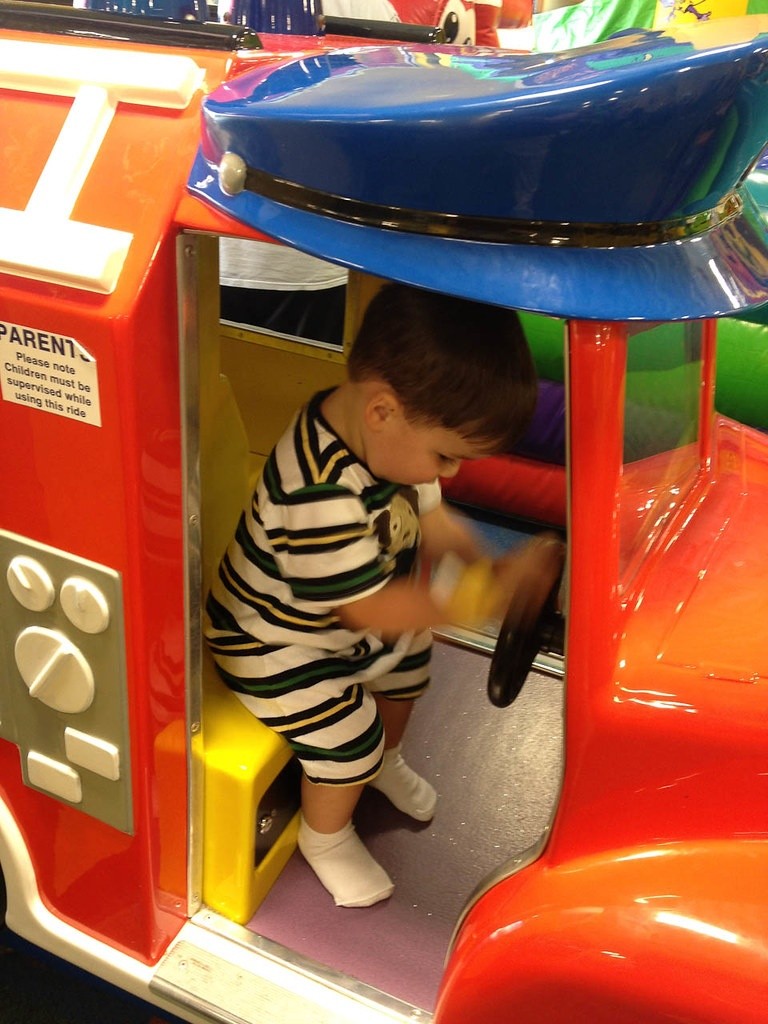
xmin=202 ymin=283 xmax=559 ymax=906
xmin=217 ymin=0 xmax=400 ymax=346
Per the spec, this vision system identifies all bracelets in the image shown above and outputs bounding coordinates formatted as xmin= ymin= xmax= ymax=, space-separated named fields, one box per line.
xmin=449 ymin=559 xmax=492 ymax=625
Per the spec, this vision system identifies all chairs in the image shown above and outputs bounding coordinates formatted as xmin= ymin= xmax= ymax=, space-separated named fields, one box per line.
xmin=196 ymin=369 xmax=302 ymax=925
